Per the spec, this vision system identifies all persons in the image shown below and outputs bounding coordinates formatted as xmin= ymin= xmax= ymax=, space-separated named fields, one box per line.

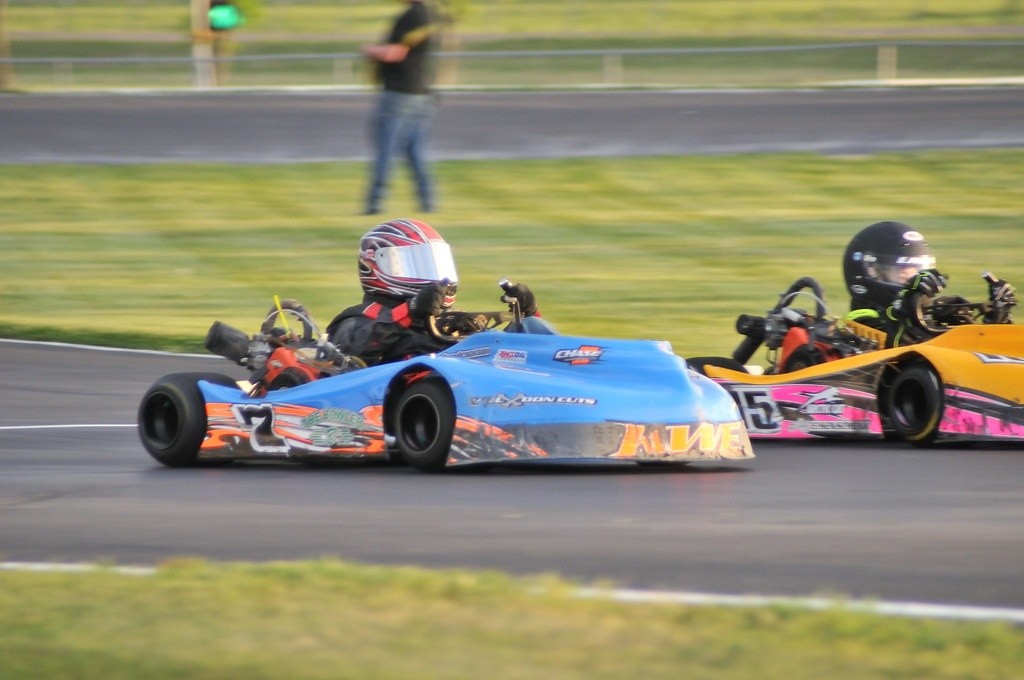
xmin=325 ymin=218 xmax=558 ymax=367
xmin=843 ymin=221 xmax=1019 ymax=348
xmin=360 ymin=0 xmax=443 ymax=214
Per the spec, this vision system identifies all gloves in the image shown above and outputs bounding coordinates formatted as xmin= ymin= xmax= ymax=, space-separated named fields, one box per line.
xmin=985 ymin=273 xmax=1024 ymax=323
xmin=407 ymin=277 xmax=450 ymax=320
xmin=902 ymin=268 xmax=950 ymax=297
xmin=498 ymin=279 xmax=537 ymax=319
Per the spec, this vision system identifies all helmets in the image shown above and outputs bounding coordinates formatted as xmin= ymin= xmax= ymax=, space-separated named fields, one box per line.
xmin=357 ymin=221 xmax=459 ymax=309
xmin=844 ymin=221 xmax=933 ymax=302
